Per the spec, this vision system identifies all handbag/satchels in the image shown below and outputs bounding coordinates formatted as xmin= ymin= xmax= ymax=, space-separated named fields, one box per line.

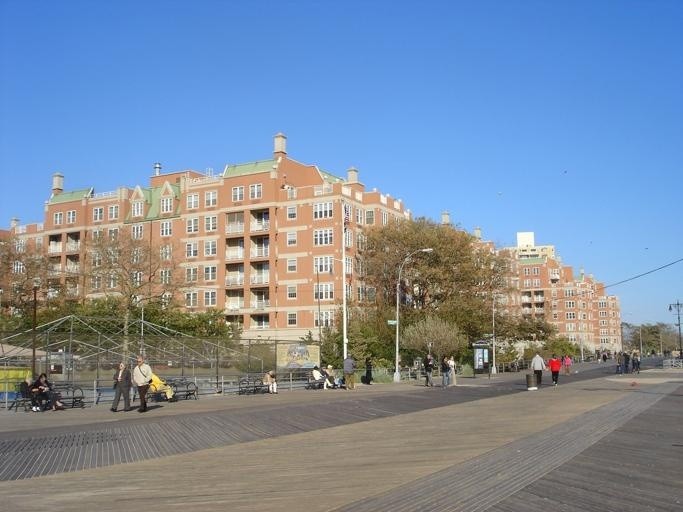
xmin=148 ymin=373 xmax=163 ymax=394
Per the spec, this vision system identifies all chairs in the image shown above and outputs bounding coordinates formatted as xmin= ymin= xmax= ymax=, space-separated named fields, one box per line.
xmin=9 ymin=384 xmax=38 ymax=412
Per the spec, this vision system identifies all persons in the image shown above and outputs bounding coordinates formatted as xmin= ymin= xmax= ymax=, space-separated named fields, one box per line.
xmin=311 ymin=364 xmax=345 ymax=390
xmin=19 ymin=376 xmax=40 ymax=412
xmin=545 ymin=353 xmax=562 ymax=386
xmin=530 ymin=351 xmax=545 ymax=386
xmin=108 ymin=362 xmax=131 ymax=412
xmin=563 ymin=354 xmax=571 ymax=375
xmin=261 ymin=370 xmax=278 ymax=394
xmin=650 ymin=348 xmax=655 ymax=359
xmin=422 ymin=354 xmax=457 ymax=390
xmin=599 ymin=348 xmax=641 ymax=374
xmin=365 ymin=356 xmax=373 ymax=385
xmin=663 ymin=349 xmax=671 ymax=358
xmin=342 ymin=354 xmax=357 ymax=390
xmin=132 ymin=353 xmax=152 ymax=413
xmin=34 ymin=372 xmax=63 ymax=411
xmin=149 ymin=372 xmax=178 ymax=402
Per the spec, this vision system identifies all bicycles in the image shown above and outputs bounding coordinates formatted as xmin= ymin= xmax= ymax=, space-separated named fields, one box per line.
xmin=632 ymin=364 xmax=640 ymax=376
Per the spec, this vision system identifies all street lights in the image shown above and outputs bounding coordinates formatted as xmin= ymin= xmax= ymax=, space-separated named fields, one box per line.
xmin=28 ymin=274 xmax=42 ymax=383
xmin=667 ymin=300 xmax=683 ymax=359
xmin=394 ymin=247 xmax=434 ymax=381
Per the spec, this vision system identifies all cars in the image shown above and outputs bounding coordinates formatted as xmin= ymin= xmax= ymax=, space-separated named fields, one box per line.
xmin=399 ymin=367 xmax=416 ymax=379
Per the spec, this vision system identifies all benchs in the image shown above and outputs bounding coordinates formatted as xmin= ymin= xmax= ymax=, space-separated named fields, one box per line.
xmin=49 ymin=382 xmax=84 ymax=409
xmin=239 ymin=378 xmax=269 ymax=393
xmin=304 ymin=375 xmax=324 ymax=389
xmin=147 ymin=382 xmax=198 ymax=402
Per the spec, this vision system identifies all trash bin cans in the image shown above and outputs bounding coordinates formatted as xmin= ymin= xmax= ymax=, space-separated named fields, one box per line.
xmin=534 ymin=370 xmax=542 ymax=386
xmin=526 ymin=374 xmax=537 ymax=391
